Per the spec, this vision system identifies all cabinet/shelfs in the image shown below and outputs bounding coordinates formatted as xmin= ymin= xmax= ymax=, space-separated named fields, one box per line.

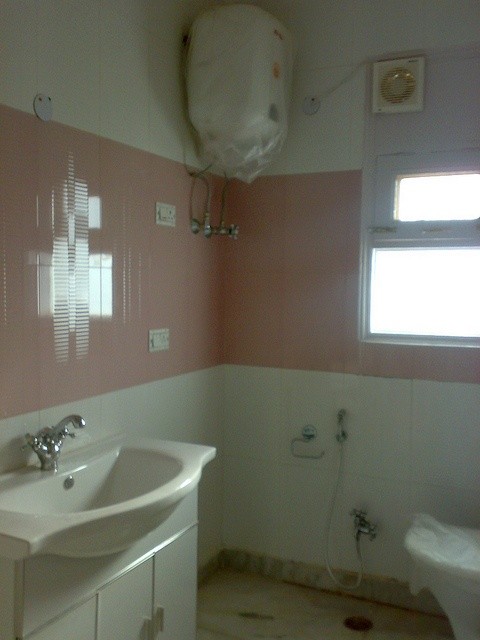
xmin=97 ymin=489 xmax=198 ymax=640
xmin=15 ymin=557 xmax=97 ymax=640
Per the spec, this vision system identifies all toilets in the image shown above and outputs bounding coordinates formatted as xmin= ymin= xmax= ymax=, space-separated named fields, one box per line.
xmin=404 ymin=522 xmax=480 ymax=640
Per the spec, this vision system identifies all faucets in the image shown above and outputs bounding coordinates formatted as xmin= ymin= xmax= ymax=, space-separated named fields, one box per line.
xmin=25 ymin=414 xmax=86 ymax=472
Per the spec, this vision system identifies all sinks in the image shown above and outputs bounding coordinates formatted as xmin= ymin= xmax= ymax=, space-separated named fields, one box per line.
xmin=0 ymin=433 xmax=216 ymax=544
